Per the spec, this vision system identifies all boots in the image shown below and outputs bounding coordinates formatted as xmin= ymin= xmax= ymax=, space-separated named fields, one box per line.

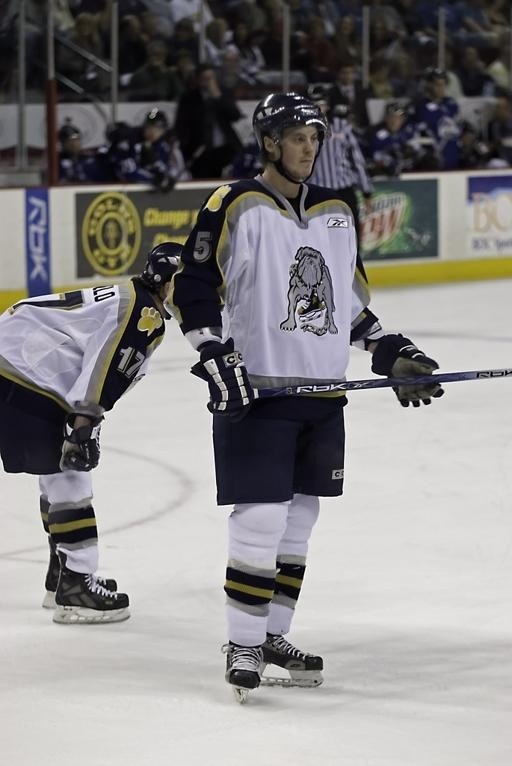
xmin=45 ymin=536 xmax=117 ymax=592
xmin=54 ymin=550 xmax=128 ymax=610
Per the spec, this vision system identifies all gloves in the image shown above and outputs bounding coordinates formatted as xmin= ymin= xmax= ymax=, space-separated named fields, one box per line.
xmin=372 ymin=333 xmax=444 ymax=407
xmin=189 ymin=337 xmax=254 ymax=423
xmin=58 ymin=414 xmax=105 ymax=471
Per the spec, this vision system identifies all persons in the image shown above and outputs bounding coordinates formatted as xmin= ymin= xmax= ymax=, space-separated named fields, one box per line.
xmin=161 ymin=86 xmax=444 ymax=692
xmin=45 ymin=0 xmax=512 ymax=196
xmin=1 ymin=240 xmax=199 ymax=614
xmin=306 ymin=75 xmax=374 ymax=288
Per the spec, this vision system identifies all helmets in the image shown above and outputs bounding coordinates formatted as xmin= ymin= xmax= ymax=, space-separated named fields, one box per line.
xmin=142 ymin=242 xmax=184 ymax=319
xmin=252 ymin=91 xmax=328 ymax=162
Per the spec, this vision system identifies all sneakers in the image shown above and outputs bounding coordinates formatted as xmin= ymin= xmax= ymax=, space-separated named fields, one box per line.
xmin=220 ymin=640 xmax=264 ymax=688
xmin=262 ymin=631 xmax=323 ymax=670
